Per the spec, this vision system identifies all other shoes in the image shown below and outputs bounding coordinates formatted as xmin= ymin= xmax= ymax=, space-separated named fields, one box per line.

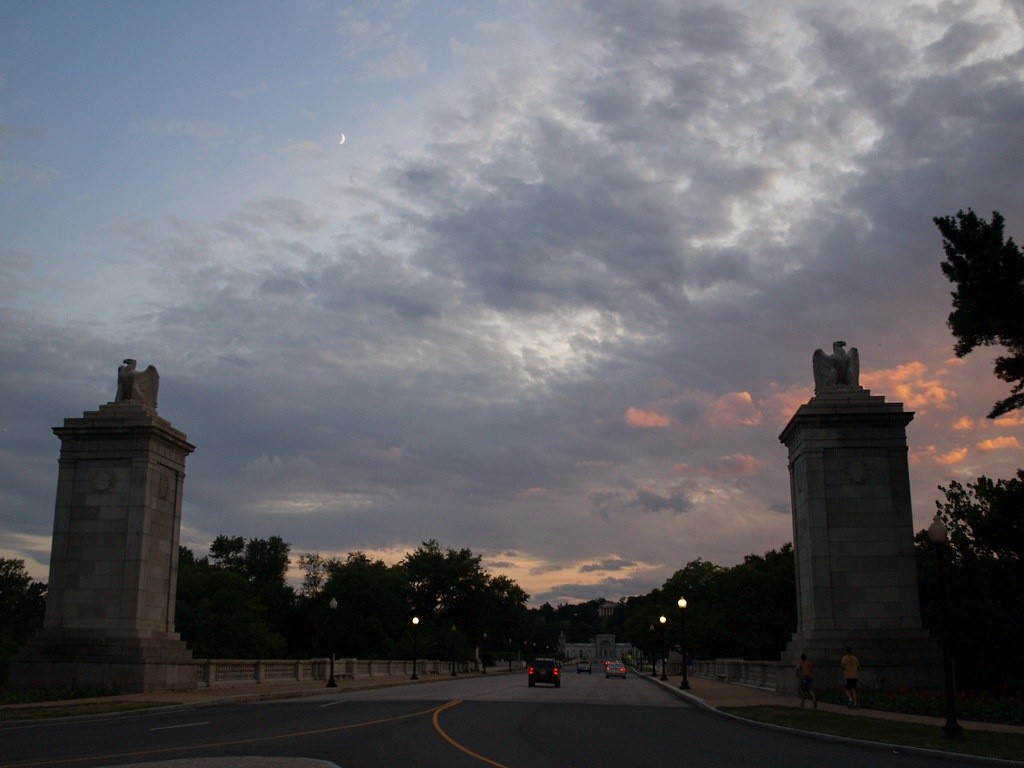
xmin=848 ymin=699 xmax=855 ymax=708
xmin=799 ymin=703 xmax=804 ymax=707
xmin=853 ymin=704 xmax=857 ymax=709
xmin=813 ymin=701 xmax=817 ymax=709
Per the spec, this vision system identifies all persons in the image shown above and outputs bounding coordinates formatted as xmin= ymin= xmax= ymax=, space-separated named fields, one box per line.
xmin=840 ymin=647 xmax=860 ymax=709
xmin=795 ymin=653 xmax=818 ymax=709
xmin=687 ymin=655 xmax=693 ymax=674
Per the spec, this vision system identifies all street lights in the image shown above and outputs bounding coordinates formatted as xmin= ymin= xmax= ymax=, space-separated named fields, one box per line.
xmin=524 ymin=641 xmax=528 ymax=669
xmin=659 ymin=614 xmax=668 ymax=681
xmin=410 ymin=617 xmax=419 ymax=679
xmin=930 ymin=514 xmax=963 ymax=733
xmin=508 ymin=638 xmax=512 ymax=672
xmin=546 ymin=645 xmax=549 ymax=658
xmin=450 ymin=625 xmax=457 ymax=675
xmin=327 ymin=600 xmax=338 ymax=688
xmin=532 ymin=643 xmax=536 ymax=661
xmin=649 ymin=624 xmax=657 ymax=677
xmin=678 ymin=596 xmax=691 ymax=690
xmin=482 ymin=632 xmax=487 ymax=674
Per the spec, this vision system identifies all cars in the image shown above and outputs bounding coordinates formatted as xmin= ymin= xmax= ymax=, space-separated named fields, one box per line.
xmin=557 ymin=661 xmax=563 ymax=668
xmin=577 ymin=660 xmax=592 ymax=674
xmin=604 ymin=659 xmax=622 ymax=671
xmin=606 ymin=662 xmax=626 ymax=679
xmin=528 ymin=658 xmax=561 ymax=688
